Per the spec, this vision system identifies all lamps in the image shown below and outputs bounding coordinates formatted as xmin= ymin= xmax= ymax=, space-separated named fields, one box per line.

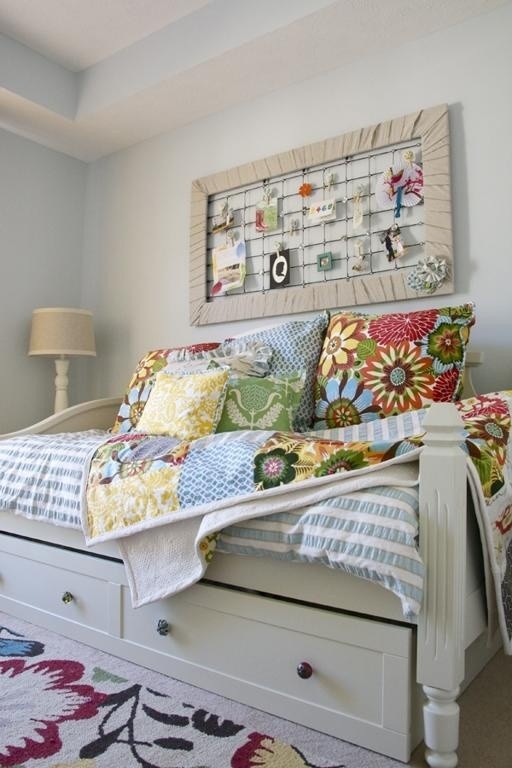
xmin=27 ymin=308 xmax=96 ymax=413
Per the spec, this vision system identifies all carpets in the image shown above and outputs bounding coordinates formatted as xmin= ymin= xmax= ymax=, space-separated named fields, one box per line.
xmin=0 ymin=609 xmax=413 ymax=768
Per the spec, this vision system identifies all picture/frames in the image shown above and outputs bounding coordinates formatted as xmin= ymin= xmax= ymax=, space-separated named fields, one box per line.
xmin=188 ymin=103 xmax=454 ymax=327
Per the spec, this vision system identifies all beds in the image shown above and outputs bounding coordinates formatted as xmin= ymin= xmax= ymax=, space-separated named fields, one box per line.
xmin=0 ymin=381 xmax=512 ymax=768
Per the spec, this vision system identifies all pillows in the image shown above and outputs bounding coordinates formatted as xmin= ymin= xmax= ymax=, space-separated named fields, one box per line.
xmin=218 ymin=372 xmax=306 ymax=435
xmin=159 ymin=343 xmax=273 ymax=377
xmin=312 ymin=299 xmax=477 ymax=431
xmin=113 ymin=342 xmax=227 ymax=435
xmin=217 ymin=308 xmax=330 ymax=428
xmin=130 ymin=367 xmax=229 ymax=439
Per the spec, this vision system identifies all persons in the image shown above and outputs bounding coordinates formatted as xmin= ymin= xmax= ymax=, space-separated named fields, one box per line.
xmin=383 ymin=231 xmax=398 ymax=262
xmin=395 ymin=234 xmax=404 ymax=253
